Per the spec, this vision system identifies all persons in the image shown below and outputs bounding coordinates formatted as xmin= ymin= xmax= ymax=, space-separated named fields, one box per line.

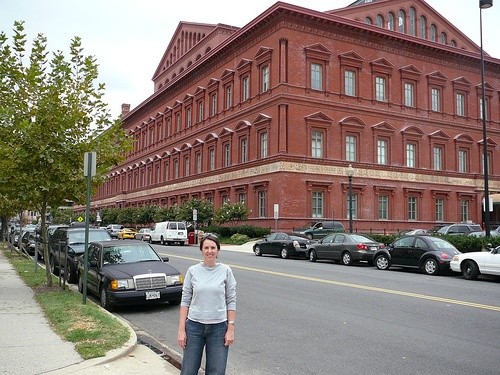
xmin=178 ymin=235 xmax=236 ymax=375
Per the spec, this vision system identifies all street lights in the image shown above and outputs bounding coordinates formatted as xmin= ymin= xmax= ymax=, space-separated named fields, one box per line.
xmin=345 ymin=164 xmax=355 ymax=234
xmin=478 ymin=0 xmax=494 ymax=239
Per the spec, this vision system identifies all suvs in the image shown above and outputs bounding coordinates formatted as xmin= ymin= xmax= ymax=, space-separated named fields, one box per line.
xmin=48 ymin=227 xmax=114 ymax=284
xmin=149 ymin=221 xmax=188 ymax=246
xmin=438 ymin=222 xmax=485 ymax=236
xmin=35 ymin=224 xmax=69 ymax=261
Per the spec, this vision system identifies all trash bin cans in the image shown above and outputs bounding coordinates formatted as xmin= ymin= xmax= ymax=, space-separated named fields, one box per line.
xmin=188 ymin=232 xmax=194 ymax=244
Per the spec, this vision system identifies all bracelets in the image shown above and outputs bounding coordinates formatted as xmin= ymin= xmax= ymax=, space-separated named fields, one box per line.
xmin=228 ymin=321 xmax=234 ymax=324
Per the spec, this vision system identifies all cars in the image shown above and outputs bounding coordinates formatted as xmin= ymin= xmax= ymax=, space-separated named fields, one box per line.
xmin=117 ymin=228 xmax=137 ymax=240
xmin=469 ymin=223 xmax=500 ymax=238
xmin=77 ymin=241 xmax=185 ymax=312
xmin=6 ymin=221 xmax=37 ymax=247
xmin=103 ymin=224 xmax=125 ymax=238
xmin=372 ymin=234 xmax=461 ymax=275
xmin=405 ymin=229 xmax=427 ymax=235
xmin=305 ymin=233 xmax=385 ymax=266
xmin=451 ymin=243 xmax=500 ymax=280
xmin=134 ymin=228 xmax=152 ymax=242
xmin=22 ymin=231 xmax=37 ymax=256
xmin=70 ymin=221 xmax=107 ymax=230
xmin=292 ymin=220 xmax=345 ymax=240
xmin=252 ymin=233 xmax=309 ymax=260
